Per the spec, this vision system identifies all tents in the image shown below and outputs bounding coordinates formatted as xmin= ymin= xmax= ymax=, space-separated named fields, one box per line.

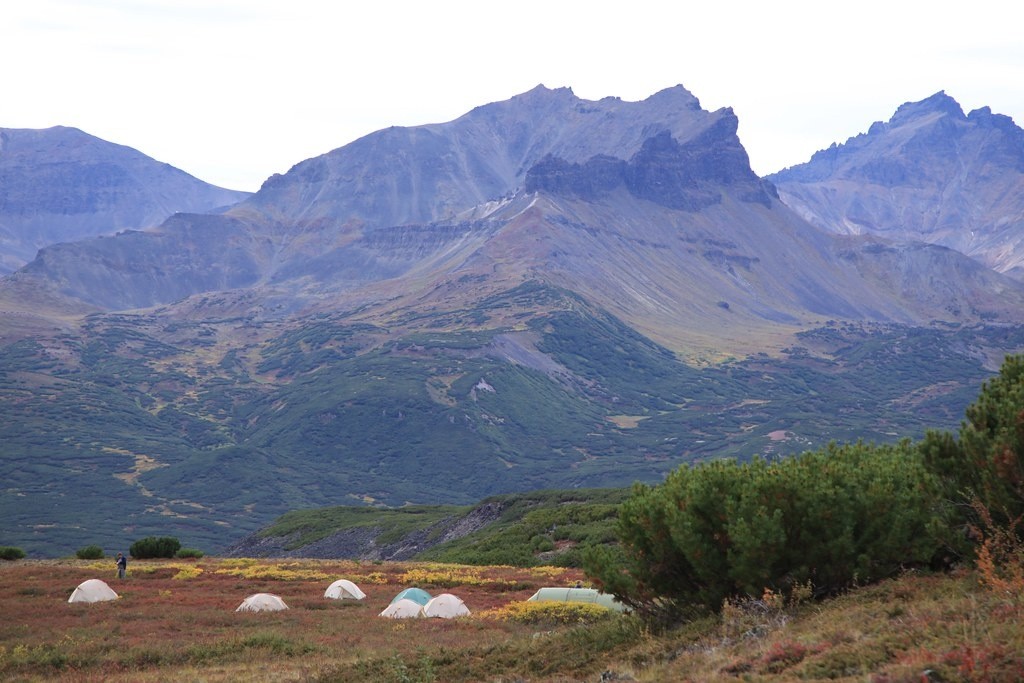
xmin=526 ymin=585 xmax=635 ymax=614
xmin=68 ymin=579 xmax=119 ymax=603
xmin=376 ymin=586 xmax=473 ymax=618
xmin=235 ymin=593 xmax=290 ymax=613
xmin=323 ymin=579 xmax=367 ymax=600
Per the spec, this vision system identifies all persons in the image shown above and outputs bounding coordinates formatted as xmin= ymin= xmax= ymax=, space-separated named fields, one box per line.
xmin=116 ymin=552 xmax=127 ymax=580
xmin=575 ymin=580 xmax=583 ymax=588
